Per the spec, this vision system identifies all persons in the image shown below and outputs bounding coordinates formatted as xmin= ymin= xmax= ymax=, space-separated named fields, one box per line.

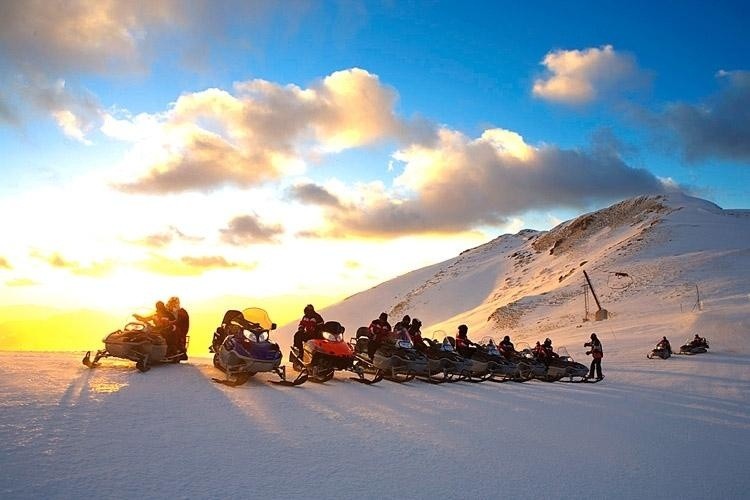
xmin=694 ymin=334 xmax=700 ymax=340
xmin=140 ymin=297 xmax=189 ymax=355
xmin=294 ymin=304 xmax=324 ymax=358
xmin=541 ymin=338 xmax=553 ymax=351
xmin=656 ymin=336 xmax=669 ymax=348
xmin=498 ymin=336 xmax=514 ymax=356
xmin=584 ymin=333 xmax=603 ymax=378
xmin=367 ymin=313 xmax=422 ymax=359
xmin=456 ymin=325 xmax=474 ymax=350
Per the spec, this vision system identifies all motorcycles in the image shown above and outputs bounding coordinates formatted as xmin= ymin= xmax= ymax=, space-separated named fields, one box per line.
xmin=82 ymin=320 xmax=190 ymax=371
xmin=674 ymin=338 xmax=710 ymax=356
xmin=647 ymin=342 xmax=672 ymax=360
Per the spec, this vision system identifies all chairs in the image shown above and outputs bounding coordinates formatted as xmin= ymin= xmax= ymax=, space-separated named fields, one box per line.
xmin=443 ymin=336 xmax=455 ymax=348
xmin=222 ymin=310 xmax=244 ymax=329
xmin=324 ymin=320 xmax=370 ymax=344
